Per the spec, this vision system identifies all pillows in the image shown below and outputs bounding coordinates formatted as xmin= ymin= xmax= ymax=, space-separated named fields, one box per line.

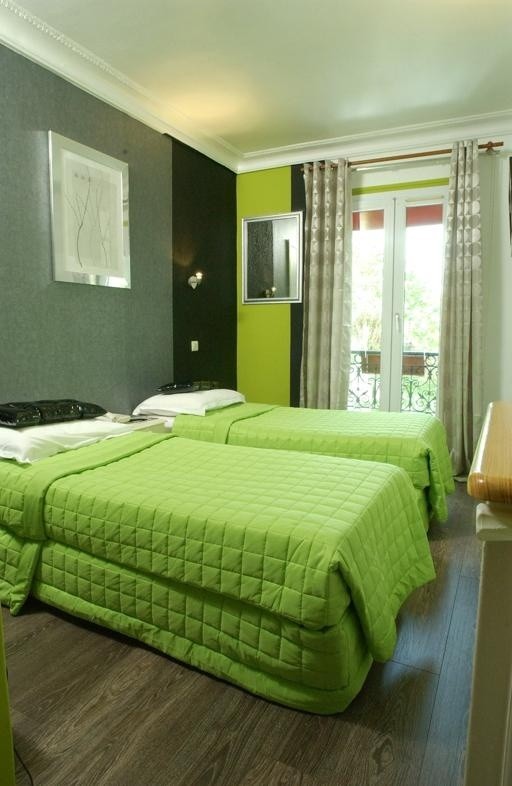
xmin=132 ymin=380 xmax=244 ymax=416
xmin=0 ymin=398 xmax=131 ymax=464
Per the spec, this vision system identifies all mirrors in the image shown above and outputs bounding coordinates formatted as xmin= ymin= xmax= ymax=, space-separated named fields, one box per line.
xmin=241 ymin=211 xmax=303 ymax=305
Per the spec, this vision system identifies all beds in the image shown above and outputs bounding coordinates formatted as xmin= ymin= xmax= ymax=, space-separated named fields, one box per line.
xmin=0 ymin=431 xmax=437 ymax=714
xmin=125 ymin=397 xmax=456 ymax=535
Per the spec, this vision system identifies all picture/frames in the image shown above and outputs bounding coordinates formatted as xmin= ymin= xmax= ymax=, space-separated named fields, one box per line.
xmin=48 ymin=130 xmax=132 ymax=289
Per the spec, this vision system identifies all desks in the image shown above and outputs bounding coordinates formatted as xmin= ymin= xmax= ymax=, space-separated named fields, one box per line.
xmin=463 ymin=399 xmax=512 ymax=786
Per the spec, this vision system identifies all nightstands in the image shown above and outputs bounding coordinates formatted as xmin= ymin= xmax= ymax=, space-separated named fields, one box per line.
xmin=105 ymin=414 xmax=168 ymax=432
